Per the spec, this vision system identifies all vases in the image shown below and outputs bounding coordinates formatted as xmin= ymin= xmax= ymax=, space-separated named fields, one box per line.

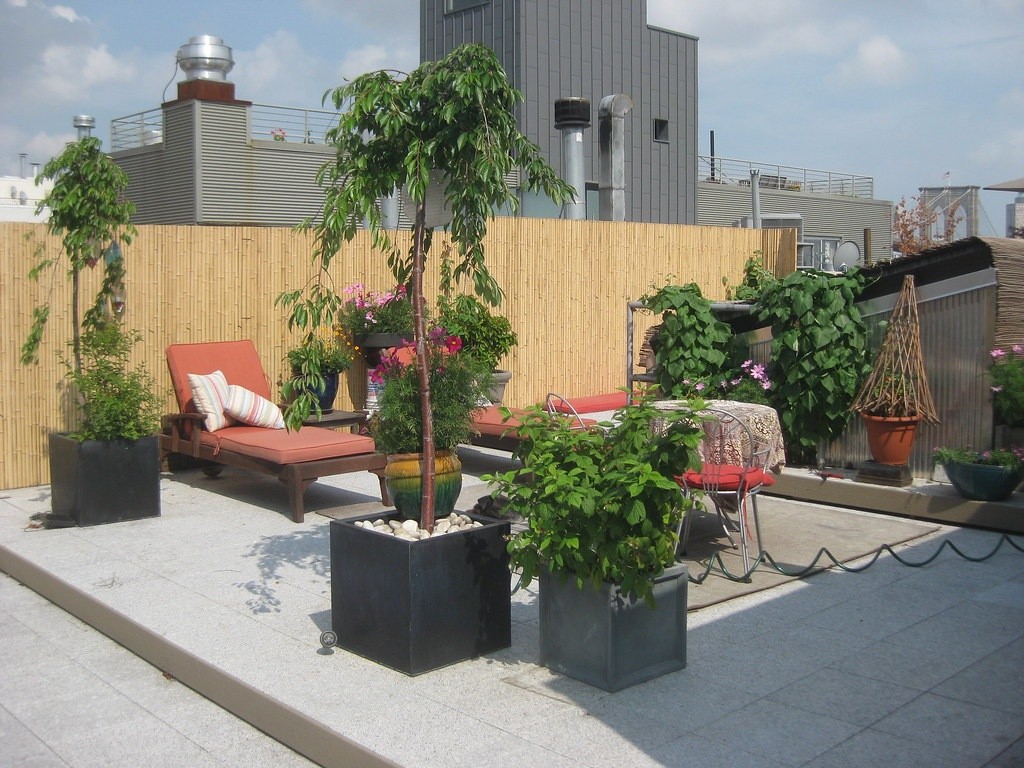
xmin=275 ymin=135 xmax=285 ymax=142
xmin=360 ymin=333 xmax=413 ymax=347
xmin=295 ymin=371 xmax=340 ymax=408
xmin=385 ymin=450 xmax=463 ymax=520
xmin=941 ymin=460 xmax=1024 ymax=500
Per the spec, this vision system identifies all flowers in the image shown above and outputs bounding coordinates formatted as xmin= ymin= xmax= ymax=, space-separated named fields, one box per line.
xmin=285 ymin=323 xmax=360 ymax=373
xmin=271 ymin=128 xmax=288 ymax=142
xmin=361 ymin=325 xmax=501 ymax=453
xmin=344 ymin=283 xmax=432 ymax=333
xmin=932 ymin=444 xmax=1024 ymax=480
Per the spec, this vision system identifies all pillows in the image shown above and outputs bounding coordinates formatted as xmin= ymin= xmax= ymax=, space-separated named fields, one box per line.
xmin=188 ymin=369 xmax=238 ymax=432
xmin=223 ymin=385 xmax=286 ymax=429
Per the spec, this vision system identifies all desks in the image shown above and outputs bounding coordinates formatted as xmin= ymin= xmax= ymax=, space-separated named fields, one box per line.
xmin=300 ymin=410 xmax=367 ymax=434
xmin=647 ymin=399 xmax=787 ymax=550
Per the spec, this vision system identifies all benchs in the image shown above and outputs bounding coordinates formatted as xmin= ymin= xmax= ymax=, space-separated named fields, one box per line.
xmin=541 ymin=390 xmax=642 ymax=434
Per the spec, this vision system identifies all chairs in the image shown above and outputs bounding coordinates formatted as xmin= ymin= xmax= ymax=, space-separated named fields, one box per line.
xmin=152 ymin=339 xmax=395 ymax=524
xmin=546 ymin=393 xmax=610 ymax=453
xmin=667 ymin=409 xmax=776 ymax=583
xmin=379 ymin=344 xmax=597 ymax=462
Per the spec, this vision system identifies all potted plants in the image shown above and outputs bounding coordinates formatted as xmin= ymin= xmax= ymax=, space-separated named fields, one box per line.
xmin=435 ymin=293 xmax=518 ymax=404
xmin=858 ymin=368 xmax=923 ymax=465
xmin=20 ymin=134 xmax=180 ymax=530
xmin=291 ymin=43 xmax=580 ymax=678
xmin=480 ymin=372 xmax=720 ymax=694
